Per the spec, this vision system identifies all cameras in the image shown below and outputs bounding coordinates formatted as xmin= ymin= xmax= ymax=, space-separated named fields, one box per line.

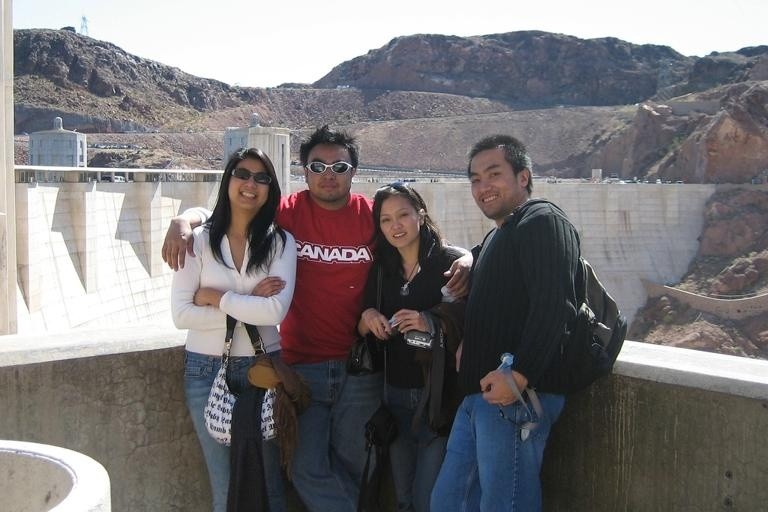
xmin=402 ymin=329 xmax=433 ymax=351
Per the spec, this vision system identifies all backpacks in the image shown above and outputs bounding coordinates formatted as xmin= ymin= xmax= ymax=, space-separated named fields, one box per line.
xmin=507 ymin=198 xmax=627 ymax=390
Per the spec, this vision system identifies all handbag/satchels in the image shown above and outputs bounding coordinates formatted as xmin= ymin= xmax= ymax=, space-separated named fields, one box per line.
xmin=203 ymin=364 xmax=278 ymax=447
xmin=346 ymin=333 xmax=385 ymax=376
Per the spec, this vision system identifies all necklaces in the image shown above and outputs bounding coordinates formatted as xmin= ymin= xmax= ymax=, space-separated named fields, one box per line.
xmin=400 ymin=261 xmax=417 ymax=297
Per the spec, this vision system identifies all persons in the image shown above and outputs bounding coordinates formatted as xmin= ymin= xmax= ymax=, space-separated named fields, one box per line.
xmin=428 ymin=134 xmax=580 ymax=512
xmin=357 ymin=182 xmax=467 ymax=512
xmin=171 ymin=148 xmax=297 ymax=512
xmin=162 ymin=124 xmax=474 ymax=512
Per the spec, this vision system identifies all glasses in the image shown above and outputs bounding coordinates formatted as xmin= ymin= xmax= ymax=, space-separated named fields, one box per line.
xmin=231 ymin=167 xmax=272 ymax=184
xmin=380 ymin=181 xmax=409 ymax=188
xmin=305 ymin=161 xmax=353 ymax=174
